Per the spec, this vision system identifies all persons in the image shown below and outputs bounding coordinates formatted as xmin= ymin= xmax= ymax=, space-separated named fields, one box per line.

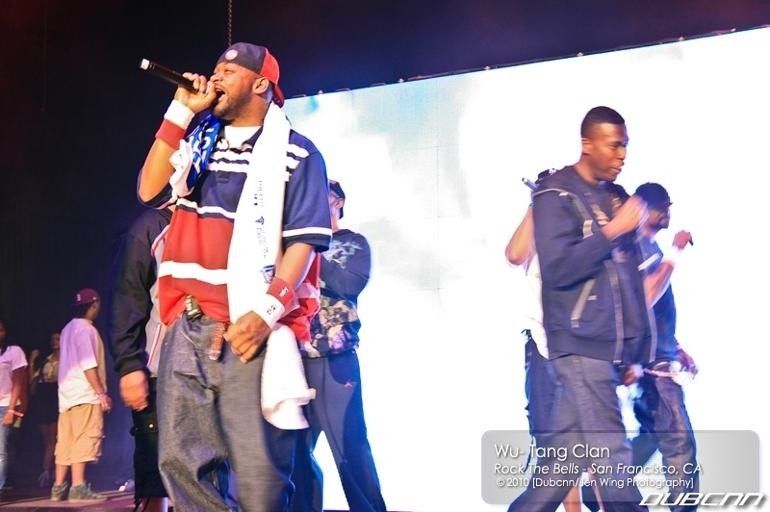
xmin=508 ymin=107 xmax=653 ymax=512
xmin=108 ymin=202 xmax=178 ymax=512
xmin=580 ymin=183 xmax=699 ymax=512
xmin=49 ymin=288 xmax=114 ymax=504
xmin=291 ymin=179 xmax=387 ymax=512
xmin=134 ymin=41 xmax=333 ymax=512
xmin=26 ymin=330 xmax=61 ymax=486
xmin=0 ymin=319 xmax=29 ymax=498
xmin=505 ymin=168 xmax=584 ymax=512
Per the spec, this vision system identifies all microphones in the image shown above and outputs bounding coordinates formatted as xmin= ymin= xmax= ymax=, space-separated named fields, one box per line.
xmin=141 ymin=57 xmax=200 ymax=96
xmin=522 ymin=175 xmax=536 ymax=189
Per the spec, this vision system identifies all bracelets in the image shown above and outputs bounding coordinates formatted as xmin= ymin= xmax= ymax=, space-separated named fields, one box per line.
xmin=154 ymin=98 xmax=196 ymax=151
xmin=6 ymin=409 xmax=16 ymax=414
xmin=97 ymin=391 xmax=106 ymax=398
xmin=251 ymin=278 xmax=296 ymax=329
xmin=27 ymin=362 xmax=34 ymax=368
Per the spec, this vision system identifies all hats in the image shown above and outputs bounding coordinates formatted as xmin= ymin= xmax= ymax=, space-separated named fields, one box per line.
xmin=633 ymin=183 xmax=672 ymax=205
xmin=216 ymin=42 xmax=284 ymax=108
xmin=71 ymin=288 xmax=100 ymax=307
xmin=329 ymin=179 xmax=345 ymax=219
xmin=535 ymin=169 xmax=560 ymax=184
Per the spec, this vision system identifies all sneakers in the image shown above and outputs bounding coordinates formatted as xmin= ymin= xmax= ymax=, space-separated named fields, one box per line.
xmin=68 ymin=481 xmax=108 ymax=504
xmin=50 ymin=480 xmax=70 ymax=502
